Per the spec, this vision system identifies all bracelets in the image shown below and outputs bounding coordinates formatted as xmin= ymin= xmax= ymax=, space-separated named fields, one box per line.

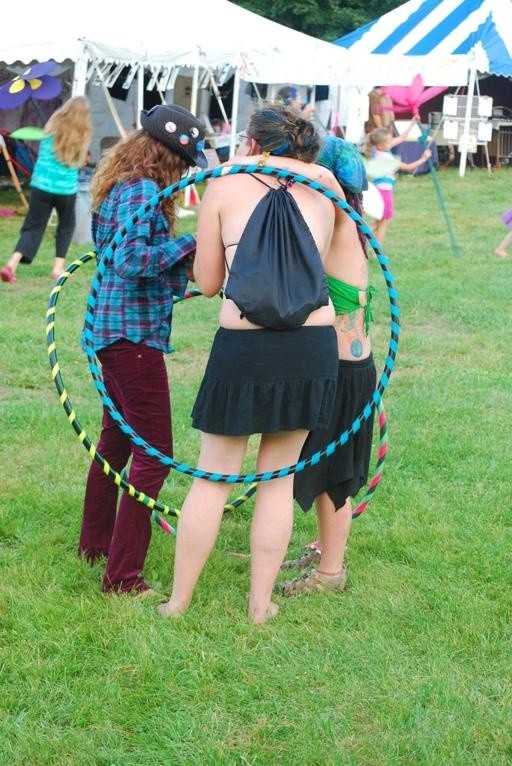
xmin=256 ymin=149 xmax=271 ymax=166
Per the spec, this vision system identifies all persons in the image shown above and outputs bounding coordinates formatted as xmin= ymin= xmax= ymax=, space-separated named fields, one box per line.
xmin=367 ymin=84 xmax=396 ymax=132
xmin=278 ymin=86 xmax=315 ymax=122
xmin=364 ymin=115 xmax=432 ymax=256
xmin=495 ymin=206 xmax=512 ymax=259
xmin=213 ymin=135 xmax=376 ymax=595
xmin=157 ymin=103 xmax=340 ymax=626
xmin=0 ymin=93 xmax=93 ymax=281
xmin=74 ymin=102 xmax=209 ymax=604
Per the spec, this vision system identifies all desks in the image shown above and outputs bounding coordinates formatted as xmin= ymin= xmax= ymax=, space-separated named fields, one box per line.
xmin=481 ymin=119 xmax=512 ymax=168
xmin=391 ymin=139 xmax=441 ymax=176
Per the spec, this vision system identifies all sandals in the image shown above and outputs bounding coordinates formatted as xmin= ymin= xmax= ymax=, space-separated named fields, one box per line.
xmin=275 ymin=563 xmax=351 ymax=600
xmin=278 ymin=543 xmax=352 ymax=569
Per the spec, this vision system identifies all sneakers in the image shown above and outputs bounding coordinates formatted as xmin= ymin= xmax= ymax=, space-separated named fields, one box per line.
xmin=0 ymin=266 xmax=18 ymax=285
xmin=50 ymin=270 xmax=74 ymax=282
xmin=127 ymin=586 xmax=171 ymax=605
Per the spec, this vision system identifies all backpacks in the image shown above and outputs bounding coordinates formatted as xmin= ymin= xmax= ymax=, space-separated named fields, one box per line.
xmin=222 ymin=185 xmax=330 ymax=332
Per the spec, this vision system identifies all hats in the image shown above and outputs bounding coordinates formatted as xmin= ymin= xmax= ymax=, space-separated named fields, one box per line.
xmin=139 ymin=102 xmax=211 ymax=171
